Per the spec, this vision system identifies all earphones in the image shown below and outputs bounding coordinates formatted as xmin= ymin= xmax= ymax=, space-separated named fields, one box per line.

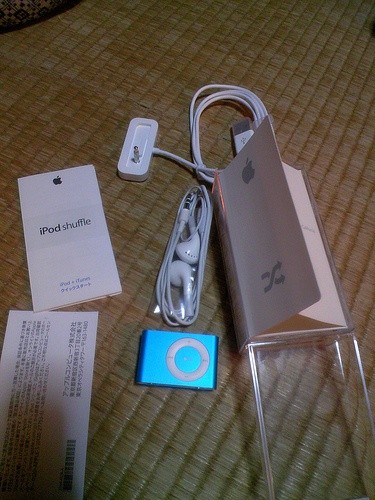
xmin=176 ymin=216 xmax=201 ymax=264
xmin=170 ymin=261 xmax=195 ymax=317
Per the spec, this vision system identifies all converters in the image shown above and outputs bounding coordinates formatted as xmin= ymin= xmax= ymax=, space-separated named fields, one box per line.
xmin=118 ymin=117 xmax=159 ymax=181
